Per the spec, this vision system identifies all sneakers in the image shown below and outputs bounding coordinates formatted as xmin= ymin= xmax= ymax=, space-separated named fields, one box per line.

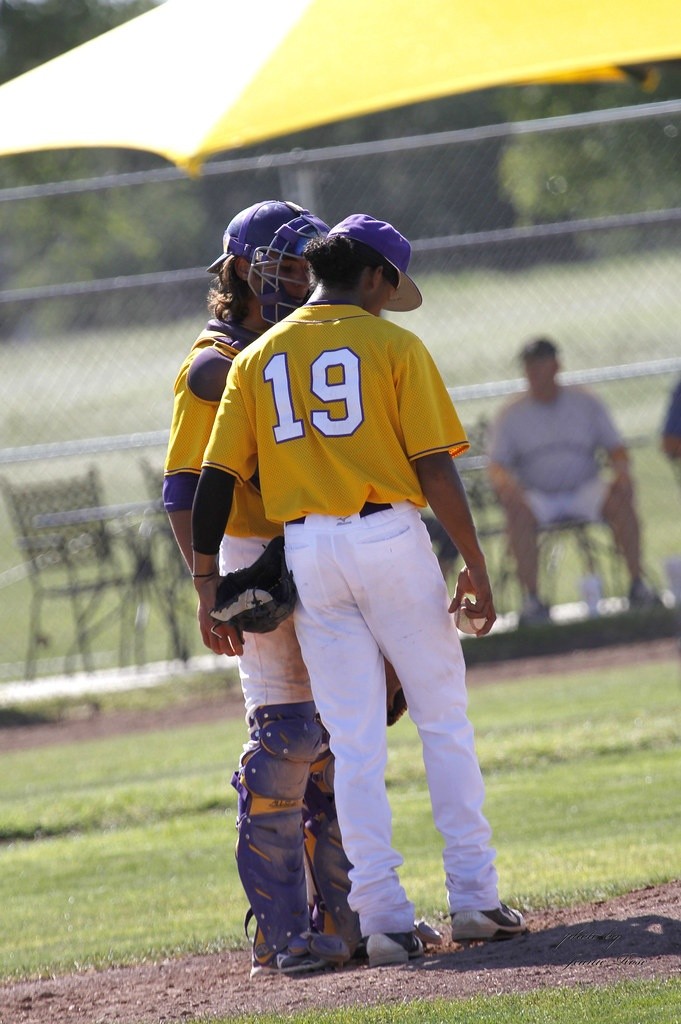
xmin=249 ymin=942 xmax=327 ymax=978
xmin=367 ymin=929 xmax=426 ymax=967
xmin=451 ymin=896 xmax=526 ymax=947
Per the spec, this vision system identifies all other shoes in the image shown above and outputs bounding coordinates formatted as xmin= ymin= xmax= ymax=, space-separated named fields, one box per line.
xmin=630 ymin=581 xmax=663 ymax=609
xmin=518 ymin=597 xmax=545 ymax=629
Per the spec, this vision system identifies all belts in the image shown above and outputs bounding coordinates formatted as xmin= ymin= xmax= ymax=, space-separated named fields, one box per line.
xmin=286 ymin=497 xmax=392 ymax=527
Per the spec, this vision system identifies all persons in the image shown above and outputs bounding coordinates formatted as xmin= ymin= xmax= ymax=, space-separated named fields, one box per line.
xmin=161 ymin=199 xmax=444 ymax=976
xmin=658 ymin=380 xmax=681 ymax=466
xmin=187 ymin=213 xmax=529 ymax=969
xmin=487 ymin=337 xmax=668 ymax=633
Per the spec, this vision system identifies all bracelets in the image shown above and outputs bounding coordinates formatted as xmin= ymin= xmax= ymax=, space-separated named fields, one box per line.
xmin=191 ymin=565 xmax=219 ymax=577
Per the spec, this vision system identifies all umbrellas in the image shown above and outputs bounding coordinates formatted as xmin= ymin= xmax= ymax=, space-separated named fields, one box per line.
xmin=0 ymin=2 xmax=681 ymax=178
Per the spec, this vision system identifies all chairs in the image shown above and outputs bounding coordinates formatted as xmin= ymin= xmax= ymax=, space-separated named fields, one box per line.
xmin=0 ymin=413 xmax=662 ymax=719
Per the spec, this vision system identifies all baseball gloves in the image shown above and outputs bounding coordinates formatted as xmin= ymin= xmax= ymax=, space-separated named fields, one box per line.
xmin=383 ymin=655 xmax=409 ymax=727
xmin=210 ymin=535 xmax=296 ymax=634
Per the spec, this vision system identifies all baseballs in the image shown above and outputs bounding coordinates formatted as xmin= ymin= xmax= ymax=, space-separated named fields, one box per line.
xmin=453 ymin=602 xmax=487 ymax=634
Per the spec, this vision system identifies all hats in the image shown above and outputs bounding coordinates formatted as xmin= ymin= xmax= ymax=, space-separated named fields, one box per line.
xmin=522 ymin=341 xmax=552 ymax=361
xmin=208 ymin=200 xmax=297 ymax=274
xmin=331 ymin=212 xmax=422 ymax=313
